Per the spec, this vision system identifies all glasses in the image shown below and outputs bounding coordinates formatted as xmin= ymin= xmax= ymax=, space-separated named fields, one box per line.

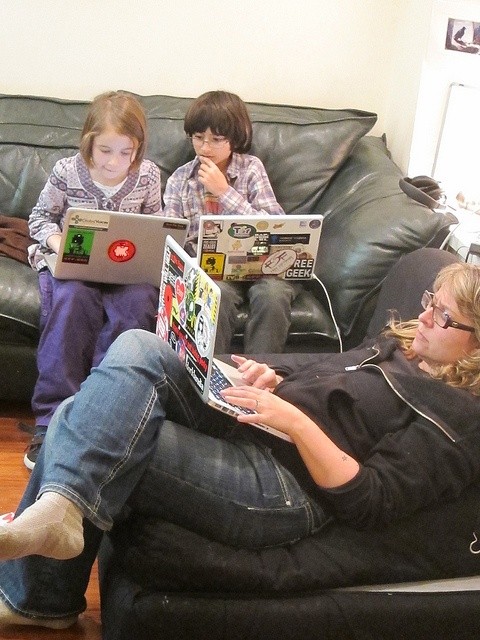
xmin=186 ymin=132 xmax=229 ymax=149
xmin=420 ymin=290 xmax=477 ymax=332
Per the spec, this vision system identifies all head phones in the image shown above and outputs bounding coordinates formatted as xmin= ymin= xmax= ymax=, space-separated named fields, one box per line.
xmin=399 ymin=176 xmax=447 ymax=210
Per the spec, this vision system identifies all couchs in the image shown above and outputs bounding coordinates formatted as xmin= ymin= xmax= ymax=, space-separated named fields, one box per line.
xmin=0 ymin=93 xmax=456 ymax=418
xmin=83 ymin=247 xmax=479 ymax=634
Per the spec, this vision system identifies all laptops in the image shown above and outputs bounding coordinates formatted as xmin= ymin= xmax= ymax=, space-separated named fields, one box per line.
xmin=41 ymin=206 xmax=192 ymax=285
xmin=156 ymin=235 xmax=295 ymax=444
xmin=191 ymin=214 xmax=325 ymax=281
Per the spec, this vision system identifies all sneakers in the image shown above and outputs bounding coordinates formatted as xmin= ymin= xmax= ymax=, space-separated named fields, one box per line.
xmin=17 ymin=422 xmax=48 ymax=470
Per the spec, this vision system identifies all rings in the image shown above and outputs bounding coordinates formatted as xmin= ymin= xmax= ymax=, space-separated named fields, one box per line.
xmin=254 ymin=400 xmax=260 ymax=411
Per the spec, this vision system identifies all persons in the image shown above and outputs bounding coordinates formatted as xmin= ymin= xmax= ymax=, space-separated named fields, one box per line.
xmin=162 ymin=90 xmax=304 ymax=354
xmin=23 ymin=91 xmax=163 ymax=471
xmin=0 ymin=262 xmax=480 ymax=629
